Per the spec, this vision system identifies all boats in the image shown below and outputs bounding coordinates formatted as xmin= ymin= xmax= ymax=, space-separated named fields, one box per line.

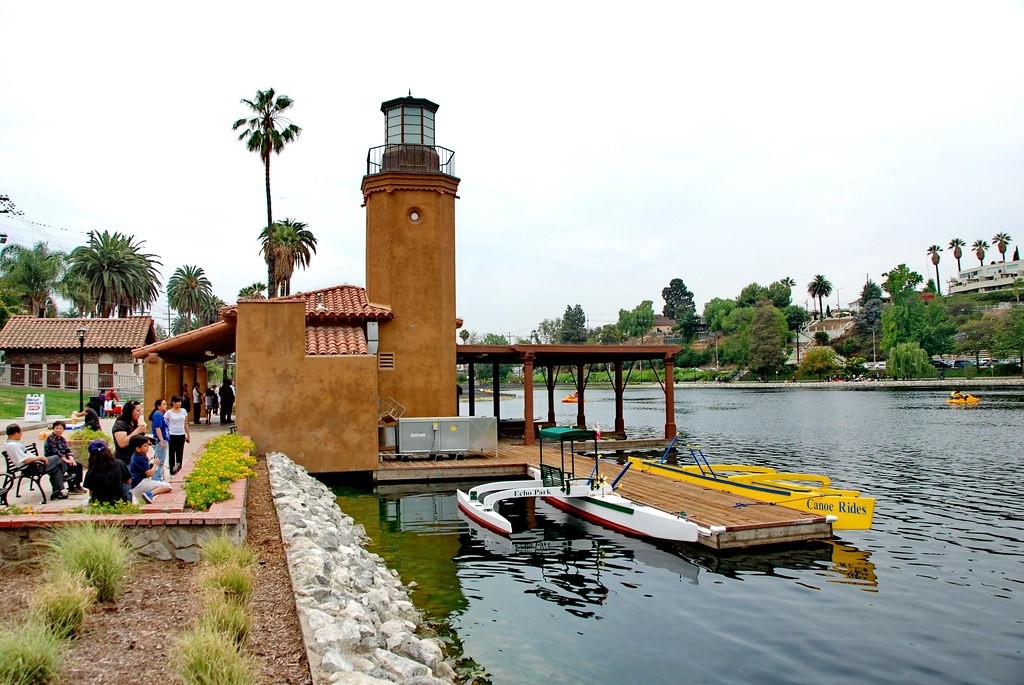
xmin=946 ymin=390 xmax=980 ymax=404
xmin=562 ymin=395 xmax=586 ymax=404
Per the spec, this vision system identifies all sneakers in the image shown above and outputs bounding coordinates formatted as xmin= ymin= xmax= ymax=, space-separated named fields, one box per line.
xmin=142 ymin=491 xmax=153 ymax=504
xmin=68 ymin=486 xmax=87 ymax=495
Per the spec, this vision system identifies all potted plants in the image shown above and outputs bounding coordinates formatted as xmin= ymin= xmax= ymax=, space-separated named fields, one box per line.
xmin=66 ymin=424 xmax=115 ymax=468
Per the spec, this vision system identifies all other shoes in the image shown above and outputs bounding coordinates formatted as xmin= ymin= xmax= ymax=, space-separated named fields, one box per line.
xmin=227 ymin=420 xmax=234 ymax=423
xmin=220 ymin=421 xmax=227 ymax=424
xmin=50 ymin=491 xmax=68 ymax=500
xmin=194 ymin=422 xmax=201 ymax=424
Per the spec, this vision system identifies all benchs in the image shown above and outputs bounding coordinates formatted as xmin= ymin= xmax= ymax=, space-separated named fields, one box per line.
xmin=0 ymin=443 xmax=76 ymax=507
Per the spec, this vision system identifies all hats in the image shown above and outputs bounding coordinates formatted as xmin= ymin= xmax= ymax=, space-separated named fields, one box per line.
xmin=88 ymin=439 xmax=108 ymax=454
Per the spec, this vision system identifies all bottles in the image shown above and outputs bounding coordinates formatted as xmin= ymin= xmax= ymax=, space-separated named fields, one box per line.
xmin=150 ymin=460 xmax=155 ymax=469
xmin=137 ymin=422 xmax=146 ymax=428
xmin=71 ymin=413 xmax=77 ymax=424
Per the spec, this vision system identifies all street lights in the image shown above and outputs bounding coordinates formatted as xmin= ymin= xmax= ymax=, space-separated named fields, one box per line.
xmin=867 ymin=327 xmax=876 ymax=364
xmin=76 ymin=329 xmax=87 ymax=413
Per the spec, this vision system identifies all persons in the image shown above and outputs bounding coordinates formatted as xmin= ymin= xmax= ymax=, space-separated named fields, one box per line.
xmin=5 ymin=420 xmax=87 ymax=501
xmin=785 ymin=378 xmax=790 ymax=383
xmin=148 ymin=395 xmax=191 ymax=482
xmin=82 ymin=400 xmax=173 ymax=510
xmin=717 ymin=377 xmax=723 ymax=383
xmin=183 ymin=378 xmax=235 ymax=425
xmin=98 ymin=387 xmax=120 ymax=419
xmin=574 ymin=392 xmax=578 ymax=398
xmin=756 ymin=376 xmax=761 ymax=383
xmin=792 ymin=375 xmax=797 ymax=382
xmin=456 ymin=378 xmax=463 ymax=416
xmin=954 ymin=390 xmax=969 ymax=401
xmin=72 ymin=402 xmax=102 ymax=432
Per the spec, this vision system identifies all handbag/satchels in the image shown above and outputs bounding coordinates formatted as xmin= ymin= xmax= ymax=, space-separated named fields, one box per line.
xmin=198 ymin=398 xmax=202 ymax=403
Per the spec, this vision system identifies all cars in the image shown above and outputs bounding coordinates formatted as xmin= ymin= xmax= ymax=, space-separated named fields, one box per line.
xmin=874 ymin=362 xmax=886 ymax=370
xmin=981 ymin=358 xmax=1002 ymax=366
xmin=930 ymin=359 xmax=953 ymax=369
xmin=954 ymin=359 xmax=976 ymax=369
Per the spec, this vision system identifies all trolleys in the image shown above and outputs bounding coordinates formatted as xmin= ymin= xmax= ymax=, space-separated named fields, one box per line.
xmin=104 ymin=398 xmax=126 ymax=419
